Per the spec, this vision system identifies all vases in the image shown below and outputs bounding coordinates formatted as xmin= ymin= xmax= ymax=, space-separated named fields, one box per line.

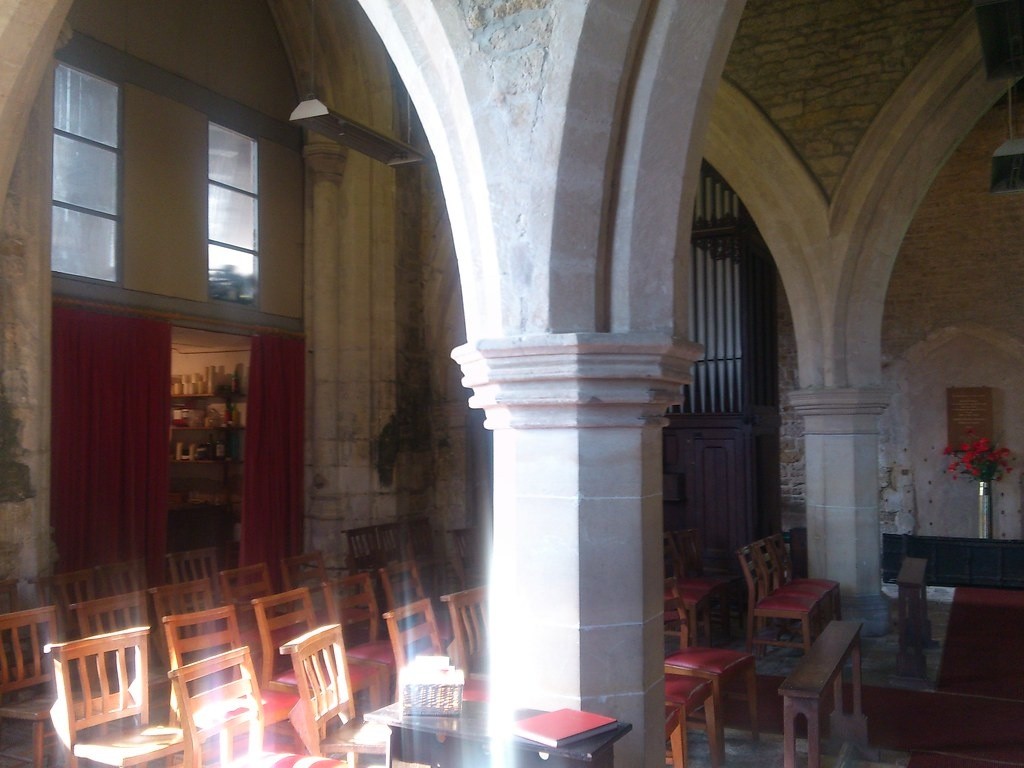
xmin=978 ymin=482 xmax=993 ymax=540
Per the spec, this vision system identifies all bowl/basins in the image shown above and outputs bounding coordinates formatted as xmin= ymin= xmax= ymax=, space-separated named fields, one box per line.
xmin=217 ymin=384 xmax=231 ymax=393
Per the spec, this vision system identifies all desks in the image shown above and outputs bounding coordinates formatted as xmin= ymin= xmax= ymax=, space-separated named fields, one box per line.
xmin=893 ymin=557 xmax=940 ymax=684
xmin=778 ymin=621 xmax=883 ymax=768
xmin=365 ymin=698 xmax=633 ymax=768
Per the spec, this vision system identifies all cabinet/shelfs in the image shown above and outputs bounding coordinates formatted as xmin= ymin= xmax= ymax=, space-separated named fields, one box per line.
xmin=881 ymin=532 xmax=1024 ymax=591
xmin=167 ymin=391 xmax=245 ymax=514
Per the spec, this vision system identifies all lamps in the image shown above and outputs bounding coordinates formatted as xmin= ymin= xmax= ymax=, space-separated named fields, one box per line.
xmin=987 ymin=77 xmax=1023 ymax=196
xmin=289 ymin=99 xmax=430 ymax=169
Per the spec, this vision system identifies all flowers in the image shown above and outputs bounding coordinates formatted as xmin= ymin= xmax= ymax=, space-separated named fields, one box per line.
xmin=944 ymin=428 xmax=1013 ymax=483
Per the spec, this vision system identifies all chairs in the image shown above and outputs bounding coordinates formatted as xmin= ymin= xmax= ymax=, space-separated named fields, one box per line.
xmin=737 ymin=533 xmax=841 ymax=654
xmin=0 ymin=519 xmax=488 ymax=768
xmin=662 ymin=529 xmax=758 ymax=768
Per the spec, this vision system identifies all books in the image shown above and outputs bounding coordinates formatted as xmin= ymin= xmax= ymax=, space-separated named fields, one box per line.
xmin=511 ymin=709 xmax=618 ymax=749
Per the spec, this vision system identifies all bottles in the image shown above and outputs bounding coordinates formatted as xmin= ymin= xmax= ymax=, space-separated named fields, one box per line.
xmin=216 ymin=439 xmax=225 ymax=460
xmin=207 ymin=433 xmax=216 ymax=460
xmin=232 ymin=367 xmax=241 ymax=393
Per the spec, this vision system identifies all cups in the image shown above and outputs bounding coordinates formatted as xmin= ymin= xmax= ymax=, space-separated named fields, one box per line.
xmin=171 ymin=365 xmax=215 ymax=394
xmin=176 ymin=442 xmax=183 ymax=460
